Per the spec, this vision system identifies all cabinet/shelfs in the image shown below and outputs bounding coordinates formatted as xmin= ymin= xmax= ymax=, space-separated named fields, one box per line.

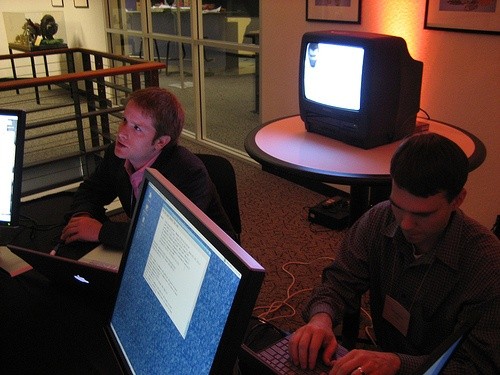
xmin=125 ymin=10 xmax=259 ymax=58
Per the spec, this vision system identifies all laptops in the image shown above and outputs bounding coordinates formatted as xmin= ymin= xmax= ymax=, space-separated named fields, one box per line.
xmin=240 ymin=331 xmax=356 ymax=375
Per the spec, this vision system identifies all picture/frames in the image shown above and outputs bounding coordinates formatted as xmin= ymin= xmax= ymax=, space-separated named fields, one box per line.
xmin=305 ymin=0 xmax=361 ymax=25
xmin=422 ymin=0 xmax=500 ymax=35
xmin=51 ymin=0 xmax=64 ymax=7
xmin=73 ymin=0 xmax=88 ymax=8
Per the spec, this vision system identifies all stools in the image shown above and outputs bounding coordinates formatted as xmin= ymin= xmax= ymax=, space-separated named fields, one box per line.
xmin=139 ymin=37 xmax=186 ymax=76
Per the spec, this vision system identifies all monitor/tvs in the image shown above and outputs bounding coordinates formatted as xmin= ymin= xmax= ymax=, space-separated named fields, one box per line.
xmin=299 ymin=30 xmax=423 ymax=149
xmin=0 ymin=109 xmax=27 ymax=226
xmin=99 ymin=167 xmax=267 ymax=375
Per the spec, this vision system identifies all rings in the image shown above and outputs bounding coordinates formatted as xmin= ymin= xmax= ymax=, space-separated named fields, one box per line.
xmin=358 ymin=366 xmax=365 ymax=375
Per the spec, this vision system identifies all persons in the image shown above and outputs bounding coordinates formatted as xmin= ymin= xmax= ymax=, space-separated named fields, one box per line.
xmin=288 ymin=133 xmax=500 ymax=375
xmin=307 ymin=43 xmax=320 ymax=68
xmin=172 ymin=0 xmax=216 ymax=10
xmin=59 ymin=86 xmax=238 ymax=248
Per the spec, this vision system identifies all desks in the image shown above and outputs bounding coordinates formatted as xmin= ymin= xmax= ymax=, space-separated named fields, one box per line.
xmin=8 ymin=42 xmax=73 ymax=104
xmin=0 ymin=213 xmax=281 ymax=375
xmin=244 ymin=113 xmax=487 ymax=226
